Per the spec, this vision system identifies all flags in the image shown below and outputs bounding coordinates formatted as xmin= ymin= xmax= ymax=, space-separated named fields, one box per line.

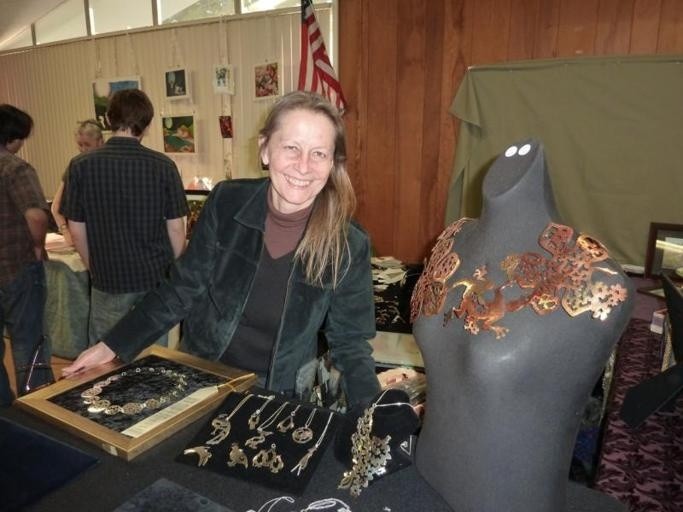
xmin=298 ymin=0 xmax=347 ymax=115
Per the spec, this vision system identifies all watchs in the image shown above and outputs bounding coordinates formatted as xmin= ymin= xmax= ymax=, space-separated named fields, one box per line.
xmin=58 ymin=223 xmax=68 ymax=233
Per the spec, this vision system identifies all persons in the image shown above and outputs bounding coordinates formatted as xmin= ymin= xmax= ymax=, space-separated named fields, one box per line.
xmin=63 ymin=88 xmax=383 ymax=415
xmin=50 ymin=121 xmax=105 ymax=352
xmin=0 ymin=103 xmax=56 ymax=409
xmin=407 ymin=134 xmax=634 ymax=511
xmin=59 ymin=89 xmax=191 ymax=350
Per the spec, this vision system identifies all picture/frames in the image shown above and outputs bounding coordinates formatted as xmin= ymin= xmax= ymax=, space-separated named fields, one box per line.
xmin=210 ymin=64 xmax=236 ymax=96
xmin=253 ymin=60 xmax=282 ymax=100
xmin=618 ymin=273 xmax=683 ymax=428
xmin=160 ymin=112 xmax=199 ymax=156
xmin=643 ymin=222 xmax=683 ymax=280
xmin=163 ymin=67 xmax=191 ymax=101
xmin=90 ymin=78 xmax=141 ymax=135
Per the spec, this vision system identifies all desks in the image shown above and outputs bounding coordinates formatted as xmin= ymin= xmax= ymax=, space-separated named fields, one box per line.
xmin=2 ymin=233 xmax=90 ymax=361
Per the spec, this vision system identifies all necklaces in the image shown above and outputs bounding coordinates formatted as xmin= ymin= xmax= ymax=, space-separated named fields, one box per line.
xmin=255 ymin=496 xmax=351 ymax=512
xmin=337 ymin=387 xmax=423 ymax=497
xmin=81 ymin=367 xmax=188 ymax=415
xmin=184 ymin=394 xmax=334 ymax=476
